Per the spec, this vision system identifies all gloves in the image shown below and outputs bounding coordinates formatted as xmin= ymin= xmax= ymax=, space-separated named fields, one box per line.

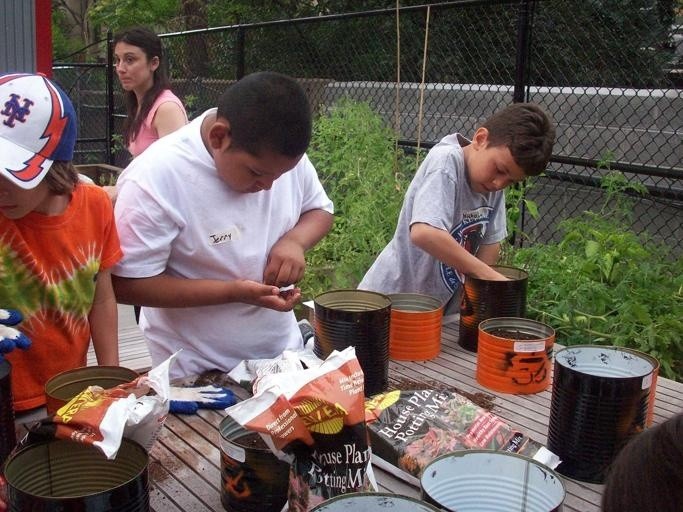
xmin=170 ymin=384 xmax=238 ymax=415
xmin=1 ymin=306 xmax=31 ymax=354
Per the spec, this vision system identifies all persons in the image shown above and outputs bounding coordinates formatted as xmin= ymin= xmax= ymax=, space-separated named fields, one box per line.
xmin=100 ymin=27 xmax=189 ymax=202
xmin=355 ymin=101 xmax=557 ymax=317
xmin=1 ymin=71 xmax=127 ymax=415
xmin=107 ymin=70 xmax=336 ymax=371
xmin=601 ymin=413 xmax=681 ymax=506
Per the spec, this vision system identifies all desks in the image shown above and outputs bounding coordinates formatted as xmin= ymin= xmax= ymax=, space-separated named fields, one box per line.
xmin=1 ymin=314 xmax=683 ymax=512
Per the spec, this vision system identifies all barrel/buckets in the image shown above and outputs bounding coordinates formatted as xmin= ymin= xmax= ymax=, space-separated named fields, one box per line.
xmin=216 ymin=414 xmax=290 ymax=512
xmin=386 ymin=293 xmax=444 ymax=361
xmin=475 ymin=318 xmax=556 ymax=392
xmin=311 ymin=289 xmax=391 ymax=399
xmin=41 ymin=364 xmax=138 ymax=415
xmin=458 ymin=263 xmax=529 ymax=354
xmin=419 ymin=448 xmax=565 ymax=512
xmin=306 ymin=491 xmax=442 ymax=512
xmin=3 ymin=435 xmax=149 ymax=508
xmin=545 ymin=345 xmax=660 ymax=486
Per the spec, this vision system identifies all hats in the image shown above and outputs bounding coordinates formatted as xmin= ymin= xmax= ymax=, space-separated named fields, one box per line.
xmin=0 ymin=73 xmax=77 ymax=191
